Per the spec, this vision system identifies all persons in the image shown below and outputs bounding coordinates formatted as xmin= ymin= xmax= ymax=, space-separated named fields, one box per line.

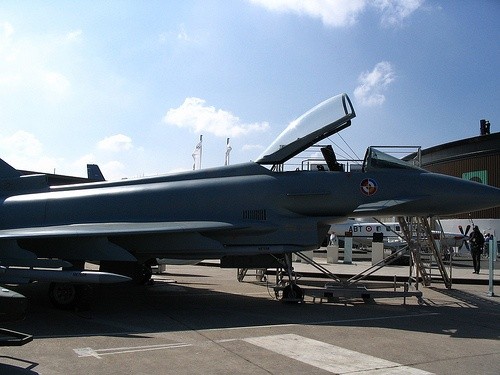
xmin=469 ymin=226 xmax=485 ymax=274
xmin=330 ymin=231 xmax=336 ymax=245
xmin=485 ymin=233 xmax=493 ymax=242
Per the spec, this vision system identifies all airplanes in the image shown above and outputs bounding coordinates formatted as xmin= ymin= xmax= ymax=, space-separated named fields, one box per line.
xmin=0 ymin=93 xmax=500 ymax=306
